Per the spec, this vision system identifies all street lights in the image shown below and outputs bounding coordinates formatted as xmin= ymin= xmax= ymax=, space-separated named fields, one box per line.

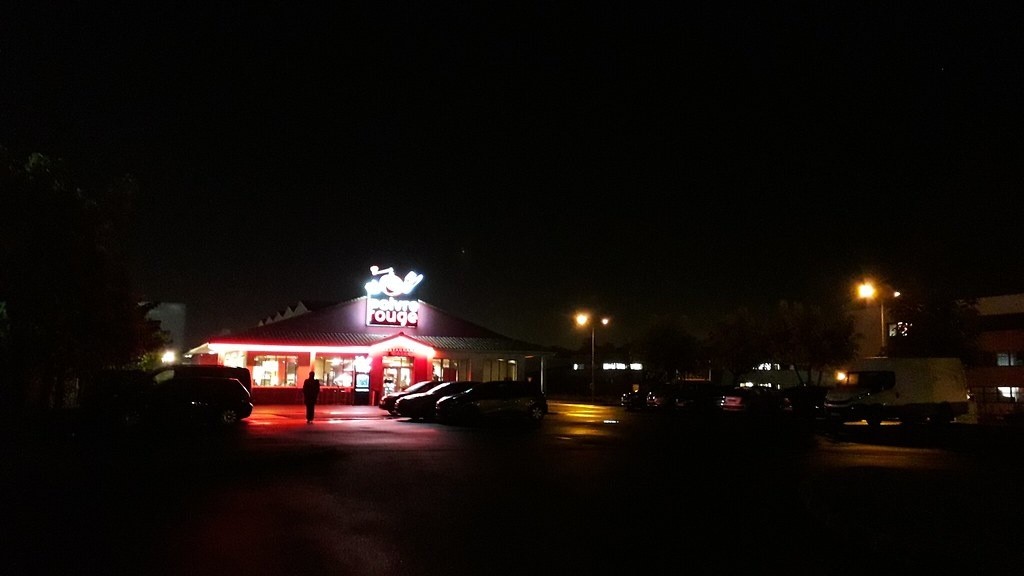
xmin=852 ymin=277 xmax=900 ymax=356
xmin=571 ymin=312 xmax=609 ymax=404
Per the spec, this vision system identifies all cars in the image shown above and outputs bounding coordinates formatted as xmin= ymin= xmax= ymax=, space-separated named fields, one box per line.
xmin=380 ymin=380 xmax=452 ymax=416
xmin=119 ymin=363 xmax=253 ymax=424
xmin=435 ymin=379 xmax=547 ymax=424
xmin=620 ymin=385 xmax=822 ymax=421
xmin=395 ymin=381 xmax=482 ymax=421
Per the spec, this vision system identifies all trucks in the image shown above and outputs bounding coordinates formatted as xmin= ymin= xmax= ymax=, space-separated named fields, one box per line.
xmin=825 ymin=356 xmax=970 ymax=428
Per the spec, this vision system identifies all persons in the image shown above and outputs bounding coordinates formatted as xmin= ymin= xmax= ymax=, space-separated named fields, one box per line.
xmin=303 ymin=371 xmax=320 ymax=422
